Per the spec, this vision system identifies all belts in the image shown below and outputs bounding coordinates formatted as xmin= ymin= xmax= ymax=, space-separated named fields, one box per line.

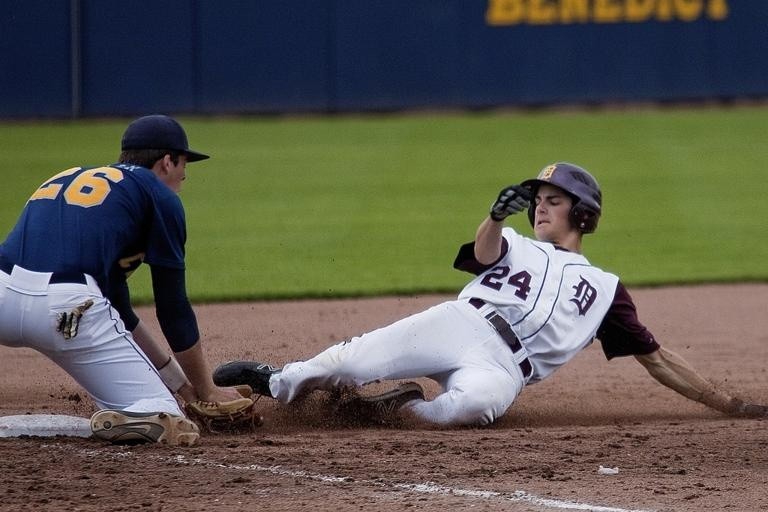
xmin=0 ymin=260 xmax=86 ymax=288
xmin=469 ymin=296 xmax=533 ymax=379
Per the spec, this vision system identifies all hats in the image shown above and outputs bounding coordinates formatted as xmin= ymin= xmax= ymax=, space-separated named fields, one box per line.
xmin=121 ymin=116 xmax=210 ymax=163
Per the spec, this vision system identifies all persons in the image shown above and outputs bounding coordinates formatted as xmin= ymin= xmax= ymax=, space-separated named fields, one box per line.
xmin=212 ymin=161 xmax=768 ymax=432
xmin=1 ymin=113 xmax=250 ymax=442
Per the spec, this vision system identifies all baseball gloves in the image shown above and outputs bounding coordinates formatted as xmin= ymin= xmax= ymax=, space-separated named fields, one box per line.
xmin=183 ymin=384 xmax=264 ymax=434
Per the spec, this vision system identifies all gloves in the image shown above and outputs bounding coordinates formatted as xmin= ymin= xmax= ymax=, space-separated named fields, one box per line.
xmin=489 ymin=185 xmax=531 ymax=222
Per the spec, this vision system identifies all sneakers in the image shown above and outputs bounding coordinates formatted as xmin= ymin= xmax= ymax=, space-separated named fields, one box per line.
xmin=92 ymin=410 xmax=200 ymax=447
xmin=211 ymin=359 xmax=283 ymax=398
xmin=346 ymin=382 xmax=425 ymax=422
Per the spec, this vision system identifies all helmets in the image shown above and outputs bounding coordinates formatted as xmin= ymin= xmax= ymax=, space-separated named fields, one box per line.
xmin=523 ymin=162 xmax=603 ymax=233
